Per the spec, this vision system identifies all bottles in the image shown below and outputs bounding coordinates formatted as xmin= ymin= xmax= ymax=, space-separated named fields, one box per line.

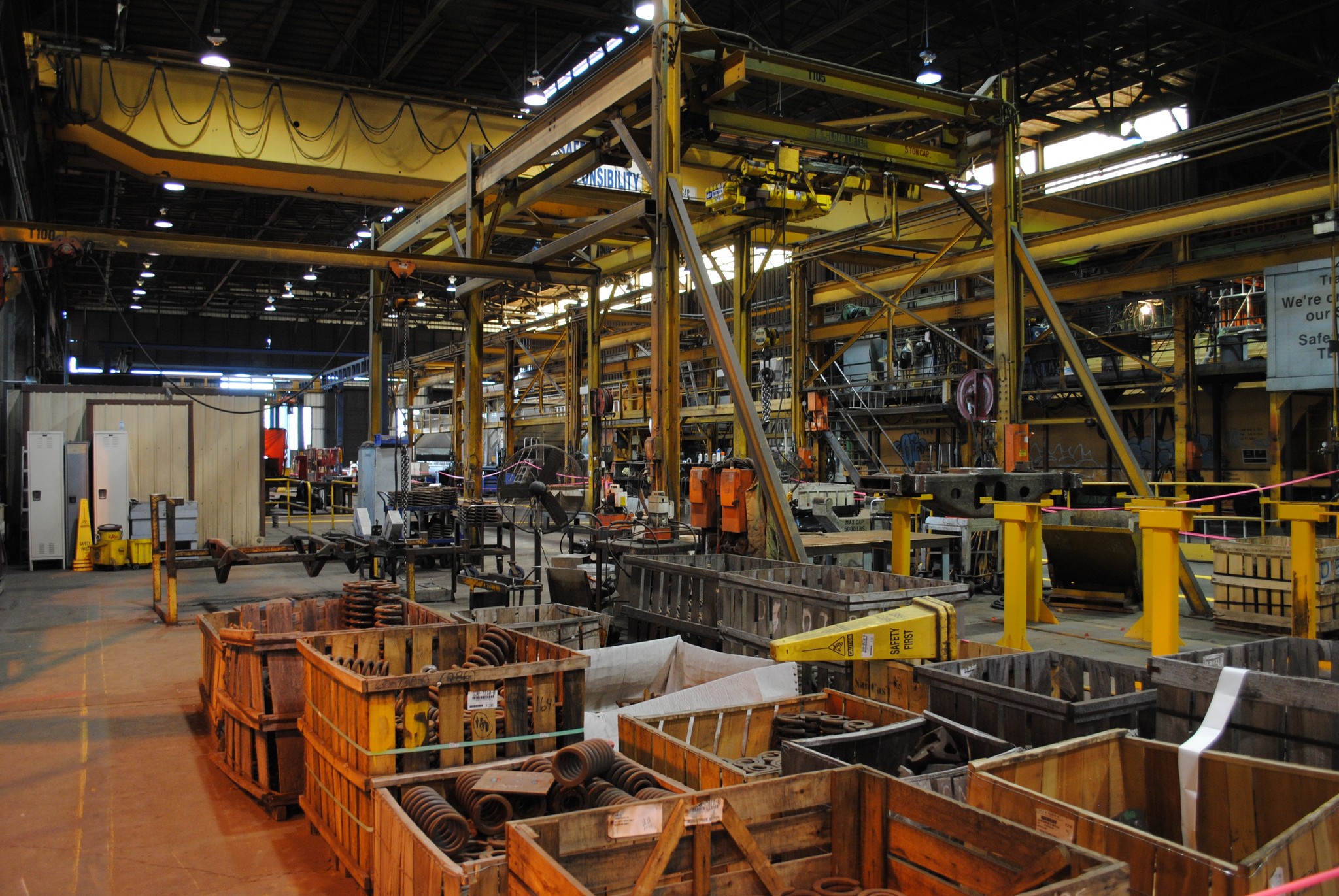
xmin=698 ymin=453 xmax=702 ymax=464
xmin=712 ymin=453 xmax=716 ymax=464
xmin=717 ymin=448 xmax=720 ymax=462
xmin=705 ymin=453 xmax=708 ymax=463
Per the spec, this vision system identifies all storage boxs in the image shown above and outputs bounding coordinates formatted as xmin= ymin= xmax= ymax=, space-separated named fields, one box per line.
xmin=547 ymin=487 xmax=584 ymax=514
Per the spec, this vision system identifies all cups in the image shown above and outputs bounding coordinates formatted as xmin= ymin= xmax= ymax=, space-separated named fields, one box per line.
xmin=721 ymin=451 xmax=725 ymax=462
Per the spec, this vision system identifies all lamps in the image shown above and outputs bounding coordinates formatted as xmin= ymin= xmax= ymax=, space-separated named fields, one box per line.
xmin=198 ymin=0 xmax=231 ymax=71
xmin=523 ymin=8 xmax=549 ymax=107
xmin=262 ymin=191 xmax=405 ymax=317
xmin=915 ymin=0 xmax=942 ymax=85
xmin=415 ymin=273 xmax=426 ymax=307
xmin=129 ymin=169 xmax=186 ymax=310
xmin=446 ymin=274 xmax=457 ymax=292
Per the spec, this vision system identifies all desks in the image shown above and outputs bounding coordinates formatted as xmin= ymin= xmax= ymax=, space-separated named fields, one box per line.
xmin=799 ymin=528 xmax=964 ymax=582
